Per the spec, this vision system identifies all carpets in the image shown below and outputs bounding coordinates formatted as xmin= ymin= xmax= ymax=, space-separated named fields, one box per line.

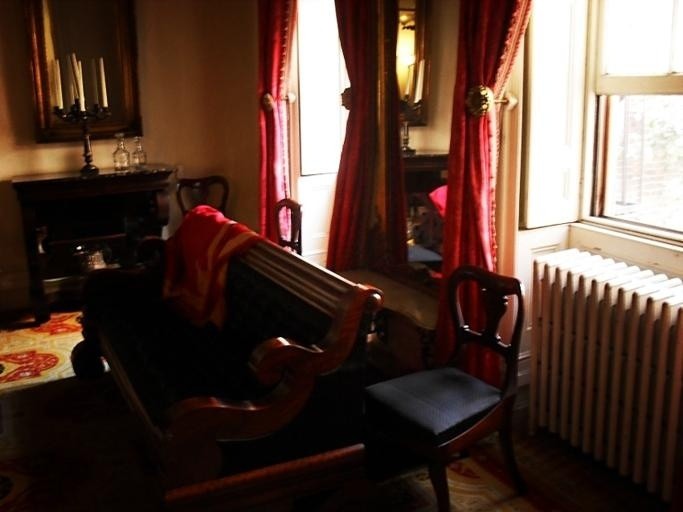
xmin=0 ymin=311 xmax=112 ymax=393
xmin=337 ymin=268 xmax=439 ymax=330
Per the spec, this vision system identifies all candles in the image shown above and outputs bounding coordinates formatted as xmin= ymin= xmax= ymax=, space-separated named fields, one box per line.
xmin=54 ymin=59 xmax=64 ymax=109
xmin=71 ymin=53 xmax=85 ymax=112
xmin=98 ymin=56 xmax=109 ymax=109
xmin=92 ymin=58 xmax=99 ymax=104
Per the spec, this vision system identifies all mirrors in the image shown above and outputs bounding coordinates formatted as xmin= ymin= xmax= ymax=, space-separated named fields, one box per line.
xmin=22 ymin=0 xmax=143 ymax=145
xmin=394 ymin=8 xmax=430 ymax=126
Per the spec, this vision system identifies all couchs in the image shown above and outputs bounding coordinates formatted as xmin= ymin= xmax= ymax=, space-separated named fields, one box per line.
xmin=71 ymin=204 xmax=384 ymax=509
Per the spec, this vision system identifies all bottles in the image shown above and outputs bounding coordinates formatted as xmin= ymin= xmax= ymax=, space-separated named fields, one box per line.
xmin=132 ymin=136 xmax=148 ymax=169
xmin=113 ymin=137 xmax=131 ymax=173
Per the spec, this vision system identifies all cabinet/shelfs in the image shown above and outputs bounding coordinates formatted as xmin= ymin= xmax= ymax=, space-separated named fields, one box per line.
xmin=11 ymin=163 xmax=177 ymax=324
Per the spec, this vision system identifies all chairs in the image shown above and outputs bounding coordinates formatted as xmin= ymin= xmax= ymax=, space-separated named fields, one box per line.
xmin=366 ymin=264 xmax=529 ymax=511
xmin=175 ymin=175 xmax=229 ymax=215
xmin=274 ymin=198 xmax=302 ymax=257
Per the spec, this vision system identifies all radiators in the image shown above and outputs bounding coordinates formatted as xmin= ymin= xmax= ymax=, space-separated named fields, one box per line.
xmin=529 ymin=247 xmax=682 ymax=511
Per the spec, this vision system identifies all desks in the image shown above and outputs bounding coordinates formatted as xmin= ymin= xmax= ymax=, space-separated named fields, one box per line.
xmin=403 ymin=152 xmax=449 ymax=246
